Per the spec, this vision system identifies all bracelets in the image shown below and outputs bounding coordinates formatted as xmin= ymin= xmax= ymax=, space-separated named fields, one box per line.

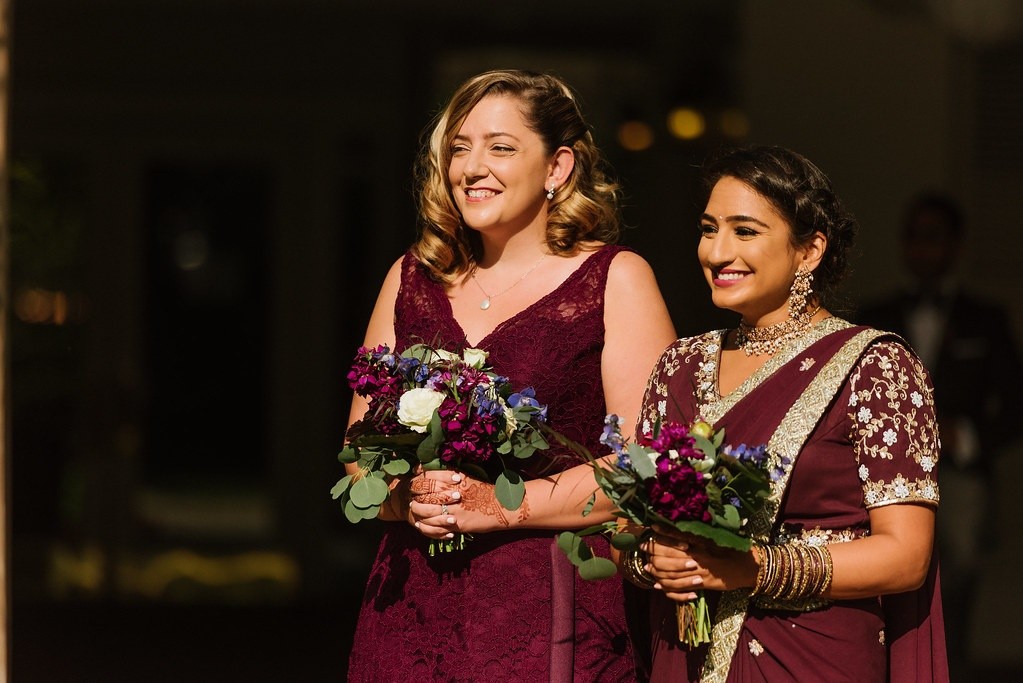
xmin=621 ymin=539 xmax=656 ymax=590
xmin=746 ymin=537 xmax=833 ymax=602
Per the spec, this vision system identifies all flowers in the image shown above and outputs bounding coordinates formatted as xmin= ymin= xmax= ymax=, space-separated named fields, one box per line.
xmin=329 ymin=327 xmax=553 ymax=558
xmin=533 ymin=359 xmax=792 ymax=652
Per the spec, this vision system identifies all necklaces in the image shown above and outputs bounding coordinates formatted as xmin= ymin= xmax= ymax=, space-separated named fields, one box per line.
xmin=467 ymin=243 xmax=554 ymax=310
xmin=734 ymin=305 xmax=819 ymax=358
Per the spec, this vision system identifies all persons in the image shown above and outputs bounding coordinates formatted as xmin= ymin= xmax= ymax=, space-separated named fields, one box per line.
xmin=344 ymin=67 xmax=679 ymax=683
xmin=632 ymin=145 xmax=953 ymax=682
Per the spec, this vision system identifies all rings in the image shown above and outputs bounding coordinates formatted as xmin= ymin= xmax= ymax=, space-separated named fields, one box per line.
xmin=442 ymin=504 xmax=448 ymax=515
